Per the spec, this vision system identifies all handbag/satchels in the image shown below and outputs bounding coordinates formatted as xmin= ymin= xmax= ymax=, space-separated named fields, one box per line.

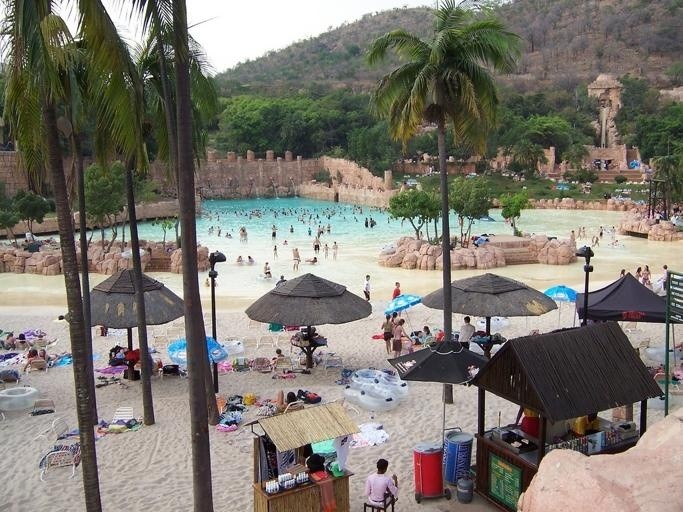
xmin=302 ymin=391 xmax=322 ymax=403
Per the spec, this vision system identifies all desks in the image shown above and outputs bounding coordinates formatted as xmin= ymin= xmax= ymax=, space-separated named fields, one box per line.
xmin=475 ymin=415 xmax=639 ymax=512
xmin=253 ymin=465 xmax=355 ymax=512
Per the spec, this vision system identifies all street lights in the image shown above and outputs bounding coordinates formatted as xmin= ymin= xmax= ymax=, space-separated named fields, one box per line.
xmin=575 ymin=246 xmax=595 ymax=324
xmin=207 ymin=251 xmax=226 ymax=393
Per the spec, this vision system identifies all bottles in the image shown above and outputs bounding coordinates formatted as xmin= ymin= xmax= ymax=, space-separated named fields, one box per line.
xmin=600 ymin=430 xmax=607 ymax=447
xmin=548 ymin=436 xmax=588 ymax=454
xmin=263 ymin=470 xmax=308 ymax=495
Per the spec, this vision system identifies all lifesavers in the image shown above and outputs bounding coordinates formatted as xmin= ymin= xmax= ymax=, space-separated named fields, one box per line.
xmin=344 ymin=368 xmax=408 ymax=411
xmin=0 ymin=387 xmax=39 ymax=411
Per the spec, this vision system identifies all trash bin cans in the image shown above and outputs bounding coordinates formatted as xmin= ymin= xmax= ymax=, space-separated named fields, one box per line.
xmin=413 ymin=444 xmax=451 ymax=504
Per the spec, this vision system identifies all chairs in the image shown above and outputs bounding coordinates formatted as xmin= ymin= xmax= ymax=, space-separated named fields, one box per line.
xmin=112 ymin=406 xmax=144 ymax=432
xmin=258 ymin=395 xmax=304 ymax=421
xmin=35 ymin=418 xmax=83 ymax=483
xmin=363 ymin=474 xmax=399 ymax=512
xmin=0 ymin=330 xmax=61 ymax=386
xmin=148 ymin=326 xmax=184 ymax=351
xmin=201 ymin=319 xmax=342 ymax=376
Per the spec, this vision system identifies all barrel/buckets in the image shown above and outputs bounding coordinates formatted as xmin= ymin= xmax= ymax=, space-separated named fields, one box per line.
xmin=413 ymin=443 xmax=444 ymax=499
xmin=444 ymin=430 xmax=474 ymax=486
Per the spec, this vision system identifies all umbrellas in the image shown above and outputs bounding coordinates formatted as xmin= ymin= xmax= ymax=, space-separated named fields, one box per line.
xmin=388 ymin=338 xmax=491 ymax=450
xmin=243 ymin=273 xmax=373 ymax=372
xmin=62 ymin=268 xmax=190 ymax=379
xmin=419 ymin=273 xmax=558 ymax=360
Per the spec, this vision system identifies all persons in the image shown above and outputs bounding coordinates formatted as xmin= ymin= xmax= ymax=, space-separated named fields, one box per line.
xmin=515 ymin=403 xmax=544 ymax=441
xmin=23 ymin=350 xmax=47 ymax=375
xmin=204 ymin=277 xmax=209 ymax=287
xmin=277 ymin=390 xmax=295 ymax=408
xmin=5 ymin=333 xmax=14 ymax=344
xmin=363 ymin=273 xmax=431 ymax=357
xmin=635 ymin=202 xmax=682 ymax=226
xmin=208 ymin=201 xmax=396 ymax=273
xmin=634 ymin=267 xmax=642 ymax=281
xmin=642 ymin=265 xmax=651 ymax=285
xmin=271 ymin=347 xmax=285 ymax=361
xmin=662 ymin=264 xmax=668 ymax=290
xmin=570 ymin=225 xmax=619 ymax=246
xmin=460 ymin=315 xmax=474 ymax=355
xmin=16 ymin=333 xmax=32 ymax=346
xmin=575 ymin=410 xmax=600 ymax=437
xmin=363 ymin=458 xmax=398 ymax=506
xmin=620 ymin=269 xmax=625 ymax=279
